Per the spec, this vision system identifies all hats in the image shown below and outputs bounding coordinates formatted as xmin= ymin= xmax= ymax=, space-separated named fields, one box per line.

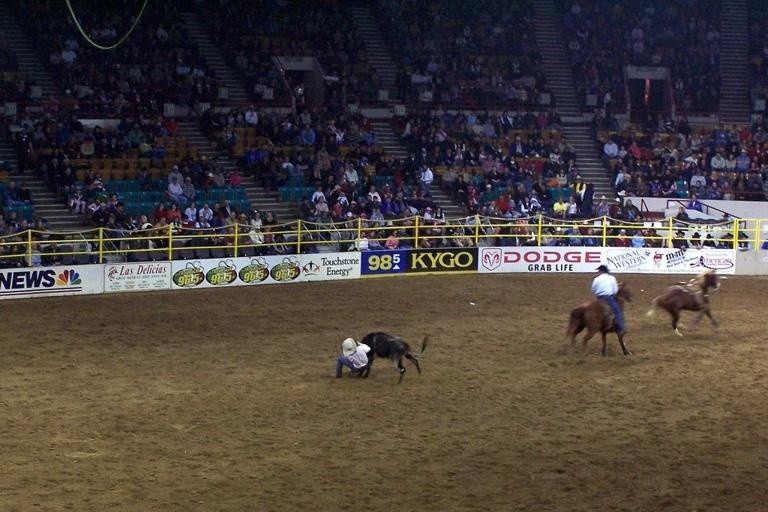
xmin=342 ymin=337 xmax=356 ymax=356
xmin=597 ymin=265 xmax=607 ymax=270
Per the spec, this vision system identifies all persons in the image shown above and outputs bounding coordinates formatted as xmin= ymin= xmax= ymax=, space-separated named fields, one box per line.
xmin=333 ymin=337 xmax=371 ymax=379
xmin=591 ymin=264 xmax=626 ymax=336
xmin=2 ymin=0 xmax=766 ymax=270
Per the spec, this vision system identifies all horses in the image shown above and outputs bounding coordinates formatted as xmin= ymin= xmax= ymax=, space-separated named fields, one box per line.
xmin=645 ymin=267 xmax=722 ymax=338
xmin=563 ymin=280 xmax=635 ymax=357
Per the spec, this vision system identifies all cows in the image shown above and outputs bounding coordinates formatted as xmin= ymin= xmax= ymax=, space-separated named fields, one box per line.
xmin=360 ymin=330 xmax=429 ymax=384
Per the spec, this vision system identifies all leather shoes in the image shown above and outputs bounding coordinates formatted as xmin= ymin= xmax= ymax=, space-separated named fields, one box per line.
xmin=329 ymin=373 xmax=336 ymax=378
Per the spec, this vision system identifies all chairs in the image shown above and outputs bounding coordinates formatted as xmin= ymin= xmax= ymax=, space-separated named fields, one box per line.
xmin=0 ymin=108 xmax=768 ymax=228
